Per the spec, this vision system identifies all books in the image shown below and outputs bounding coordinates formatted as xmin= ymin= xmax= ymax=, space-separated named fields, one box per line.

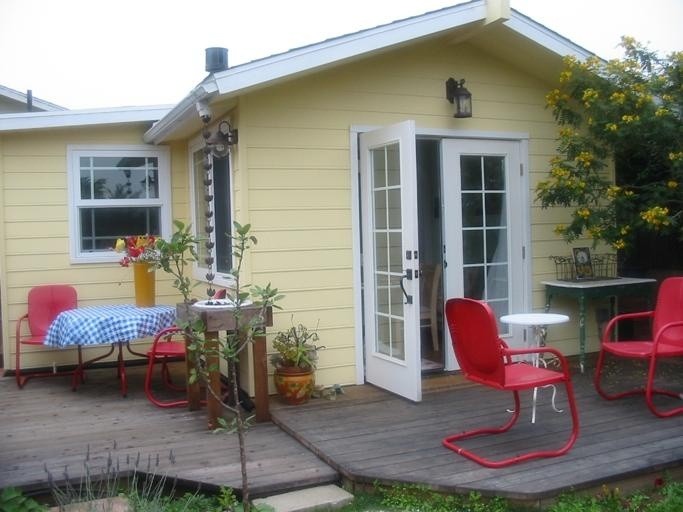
xmin=572 ymin=247 xmax=595 ymax=280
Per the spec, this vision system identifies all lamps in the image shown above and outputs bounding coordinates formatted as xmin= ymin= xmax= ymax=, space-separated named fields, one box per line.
xmin=205 ymin=120 xmax=240 ymax=160
xmin=443 ymin=69 xmax=475 ymax=121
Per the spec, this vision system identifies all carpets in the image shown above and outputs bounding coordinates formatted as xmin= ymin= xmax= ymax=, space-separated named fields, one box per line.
xmin=418 ymin=367 xmax=487 ymax=395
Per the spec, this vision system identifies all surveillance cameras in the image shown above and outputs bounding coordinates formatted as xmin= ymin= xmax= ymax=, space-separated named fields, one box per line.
xmin=197 ymin=102 xmax=212 ymax=123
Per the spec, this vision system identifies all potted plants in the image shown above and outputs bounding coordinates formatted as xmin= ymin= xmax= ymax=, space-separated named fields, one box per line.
xmin=270 ymin=311 xmax=327 ymax=406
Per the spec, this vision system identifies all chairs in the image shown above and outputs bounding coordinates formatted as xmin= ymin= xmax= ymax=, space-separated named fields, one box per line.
xmin=391 ymin=258 xmax=441 ymax=353
xmin=144 ymin=287 xmax=227 ymax=410
xmin=592 ymin=272 xmax=683 ymax=419
xmin=437 ymin=295 xmax=581 ymax=468
xmin=14 ymin=284 xmax=85 ymax=391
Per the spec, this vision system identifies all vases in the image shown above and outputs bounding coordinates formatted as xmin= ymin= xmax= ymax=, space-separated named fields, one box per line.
xmin=133 ymin=259 xmax=158 ymax=309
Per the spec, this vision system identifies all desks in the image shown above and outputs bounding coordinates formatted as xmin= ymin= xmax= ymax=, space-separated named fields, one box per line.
xmin=539 ymin=273 xmax=660 ymax=378
xmin=44 ymin=303 xmax=182 ymax=399
xmin=498 ymin=313 xmax=573 ymax=429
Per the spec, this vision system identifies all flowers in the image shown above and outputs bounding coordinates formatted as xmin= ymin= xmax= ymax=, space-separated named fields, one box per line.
xmin=112 ymin=230 xmax=168 ymax=270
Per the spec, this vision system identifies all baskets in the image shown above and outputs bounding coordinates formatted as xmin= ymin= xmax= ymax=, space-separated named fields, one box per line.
xmin=550 ymin=253 xmax=622 ymax=282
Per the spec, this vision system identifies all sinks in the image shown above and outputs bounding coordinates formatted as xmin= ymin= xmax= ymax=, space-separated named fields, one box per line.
xmin=193 ymin=298 xmax=252 ymax=309
